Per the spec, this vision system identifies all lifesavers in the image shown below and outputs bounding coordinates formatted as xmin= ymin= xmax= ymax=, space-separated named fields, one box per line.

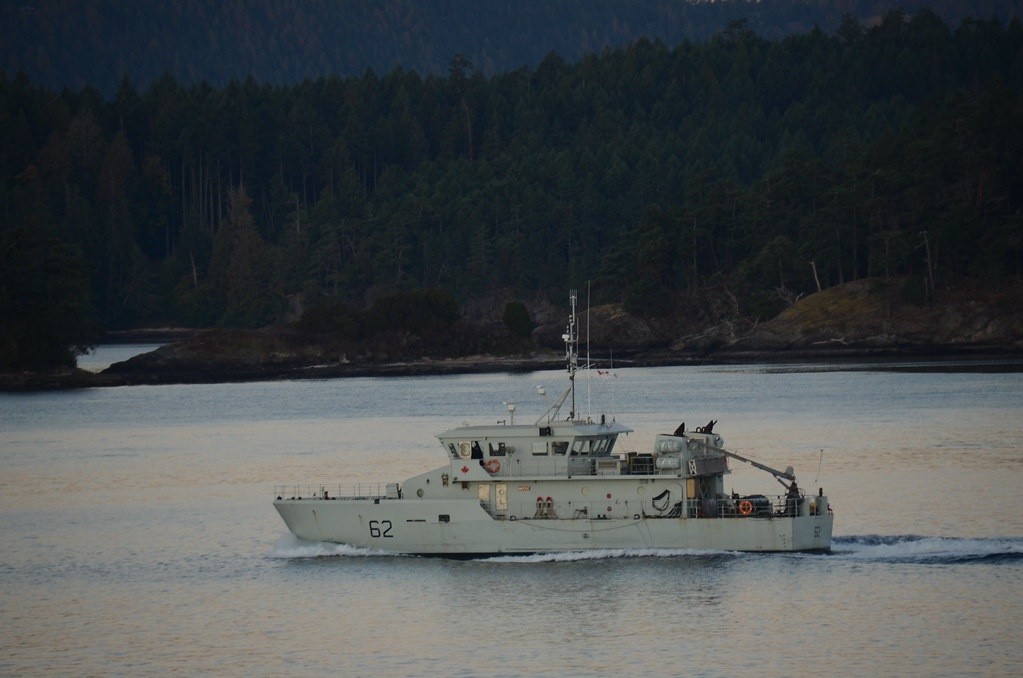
xmin=740 ymin=501 xmax=752 ymax=515
xmin=487 ymin=459 xmax=500 ymax=472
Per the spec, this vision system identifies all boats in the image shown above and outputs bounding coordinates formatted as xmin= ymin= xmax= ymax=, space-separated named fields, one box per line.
xmin=270 ymin=293 xmax=836 ymax=556
